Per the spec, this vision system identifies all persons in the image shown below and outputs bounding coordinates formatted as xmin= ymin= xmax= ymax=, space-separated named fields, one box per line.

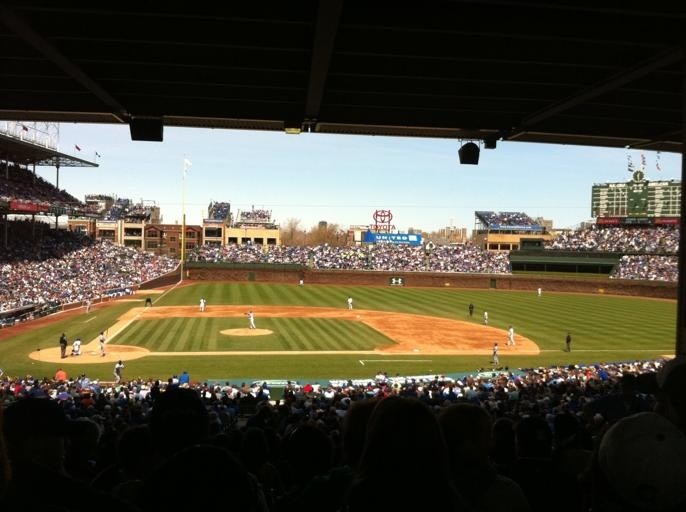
xmin=3 ymin=164 xmax=686 ymax=510
